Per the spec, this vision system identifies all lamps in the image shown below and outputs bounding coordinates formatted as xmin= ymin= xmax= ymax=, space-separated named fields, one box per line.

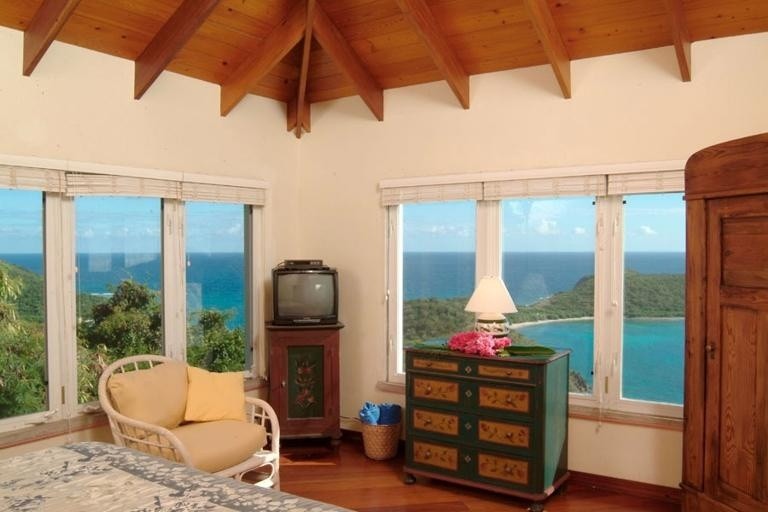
xmin=465 ymin=276 xmax=518 ymax=339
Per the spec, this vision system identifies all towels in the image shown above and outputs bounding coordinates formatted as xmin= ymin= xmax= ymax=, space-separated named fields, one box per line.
xmin=360 ymin=402 xmax=380 ymax=424
xmin=380 ymin=401 xmax=401 ymax=425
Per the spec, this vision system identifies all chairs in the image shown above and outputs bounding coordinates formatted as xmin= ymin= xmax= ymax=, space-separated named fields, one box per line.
xmin=98 ymin=354 xmax=280 ymax=489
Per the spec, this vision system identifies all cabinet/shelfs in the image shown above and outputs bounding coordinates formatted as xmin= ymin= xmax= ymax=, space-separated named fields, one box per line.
xmin=679 ymin=132 xmax=767 ymax=511
xmin=265 ymin=320 xmax=344 ymax=455
xmin=405 ymin=341 xmax=571 ymax=511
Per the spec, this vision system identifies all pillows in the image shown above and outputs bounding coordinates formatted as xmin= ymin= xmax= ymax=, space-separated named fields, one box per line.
xmin=185 ymin=364 xmax=246 ymax=422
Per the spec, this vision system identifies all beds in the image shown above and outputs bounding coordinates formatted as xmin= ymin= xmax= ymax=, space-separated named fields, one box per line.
xmin=0 ymin=438 xmax=360 ymax=511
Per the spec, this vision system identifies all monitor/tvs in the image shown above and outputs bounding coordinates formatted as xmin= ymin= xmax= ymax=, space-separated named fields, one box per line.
xmin=272 ymin=269 xmax=338 ymax=325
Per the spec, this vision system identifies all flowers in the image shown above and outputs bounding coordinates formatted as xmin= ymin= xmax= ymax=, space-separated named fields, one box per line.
xmin=448 ymin=330 xmax=513 ymax=356
